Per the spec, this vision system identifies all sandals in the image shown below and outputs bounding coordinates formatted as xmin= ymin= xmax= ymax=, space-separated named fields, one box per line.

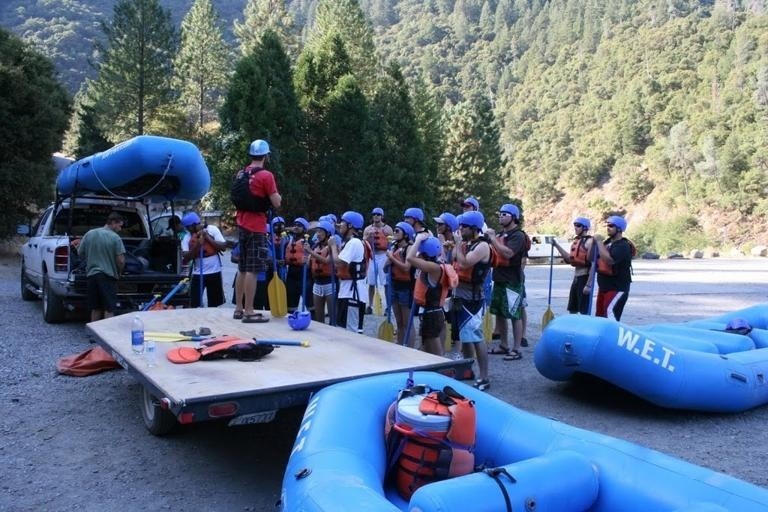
xmin=233 ymin=310 xmax=244 ymax=318
xmin=241 ymin=312 xmax=269 ymax=323
xmin=504 ymin=349 xmax=522 ymax=360
xmin=487 ymin=344 xmax=510 ymax=354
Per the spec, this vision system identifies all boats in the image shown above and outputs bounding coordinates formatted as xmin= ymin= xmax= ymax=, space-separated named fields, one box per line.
xmin=537 ymin=304 xmax=768 ymax=405
xmin=53 ymin=137 xmax=212 ymax=200
xmin=282 ymin=374 xmax=767 ymax=512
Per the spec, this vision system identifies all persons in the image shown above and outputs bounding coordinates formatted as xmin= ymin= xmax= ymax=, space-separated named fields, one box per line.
xmin=76 ymin=212 xmax=127 ymax=322
xmin=181 ymin=212 xmax=228 ymax=308
xmin=165 ymin=217 xmax=193 ymax=251
xmin=548 ymin=218 xmax=599 ymax=316
xmin=593 ymin=215 xmax=634 ymax=322
xmin=229 ymin=138 xmax=283 ymax=324
xmin=228 ymin=196 xmax=531 ymax=363
xmin=446 ymin=209 xmax=493 ymax=392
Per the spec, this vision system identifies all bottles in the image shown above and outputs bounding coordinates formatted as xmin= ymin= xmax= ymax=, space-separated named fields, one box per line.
xmin=130 ymin=315 xmax=144 ymax=354
xmin=146 ymin=337 xmax=157 ymax=368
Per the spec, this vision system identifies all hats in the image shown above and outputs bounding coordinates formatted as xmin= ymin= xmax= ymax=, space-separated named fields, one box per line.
xmin=460 ymin=202 xmax=472 ymax=206
xmin=166 ymin=215 xmax=181 ymax=230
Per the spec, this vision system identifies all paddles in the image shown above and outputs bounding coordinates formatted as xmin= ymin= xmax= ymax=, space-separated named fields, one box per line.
xmin=483 ymin=275 xmax=493 ymax=344
xmin=372 ymin=240 xmax=383 ymax=315
xmin=379 ymin=236 xmax=393 ymax=343
xmin=443 ymin=243 xmax=452 ymax=352
xmin=268 ymin=210 xmax=288 ymax=317
xmin=542 ymin=237 xmax=554 ymax=331
xmin=143 ymin=332 xmax=309 ymax=346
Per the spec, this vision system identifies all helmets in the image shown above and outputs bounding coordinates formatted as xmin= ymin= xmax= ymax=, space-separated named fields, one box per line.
xmin=271 ymin=216 xmax=285 ymax=225
xmin=340 ymin=210 xmax=364 ymax=228
xmin=294 ymin=217 xmax=309 ymax=232
xmin=464 ymin=197 xmax=480 ymax=210
xmin=288 ymin=311 xmax=312 ymax=330
xmin=500 ymin=203 xmax=520 ymax=220
xmin=439 ymin=212 xmax=459 ymax=233
xmin=403 ymin=207 xmax=423 ymax=221
xmin=455 ymin=213 xmax=463 ymax=224
xmin=606 ymin=215 xmax=627 ymax=232
xmin=327 ymin=213 xmax=337 ymax=222
xmin=372 ymin=207 xmax=384 ymax=216
xmin=249 ymin=139 xmax=271 ymax=156
xmin=574 ymin=217 xmax=590 ymax=228
xmin=418 ymin=236 xmax=442 ymax=257
xmin=459 ymin=210 xmax=485 ymax=229
xmin=182 ymin=211 xmax=200 ymax=228
xmin=395 ymin=221 xmax=415 ymax=239
xmin=316 ymin=220 xmax=334 ymax=237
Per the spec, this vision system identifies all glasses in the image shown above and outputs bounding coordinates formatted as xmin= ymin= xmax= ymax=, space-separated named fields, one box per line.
xmin=294 ymin=223 xmax=303 ymax=227
xmin=500 ymin=212 xmax=512 ymax=217
xmin=460 ymin=223 xmax=469 ymax=228
xmin=574 ymin=223 xmax=582 ymax=227
xmin=393 ymin=228 xmax=399 ymax=234
xmin=372 ymin=213 xmax=381 ymax=216
xmin=606 ymin=223 xmax=615 ymax=227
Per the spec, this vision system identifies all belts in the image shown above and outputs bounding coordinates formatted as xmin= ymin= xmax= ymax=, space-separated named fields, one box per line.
xmin=426 ymin=309 xmax=442 ymax=313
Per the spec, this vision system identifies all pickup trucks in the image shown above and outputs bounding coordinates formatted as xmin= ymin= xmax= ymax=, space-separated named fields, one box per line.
xmin=24 ymin=203 xmax=205 ymax=315
xmin=527 ymin=233 xmax=572 ymax=262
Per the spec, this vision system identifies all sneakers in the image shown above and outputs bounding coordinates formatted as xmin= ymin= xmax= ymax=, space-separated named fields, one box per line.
xmin=455 ymin=369 xmax=475 ymax=381
xmin=365 ymin=307 xmax=372 ymax=315
xmin=474 ymin=378 xmax=490 ymax=391
xmin=492 ymin=333 xmax=501 ymax=340
xmin=521 ymin=338 xmax=529 ymax=347
xmin=384 ymin=307 xmax=388 ymax=316
xmin=441 ymin=367 xmax=457 ymax=375
